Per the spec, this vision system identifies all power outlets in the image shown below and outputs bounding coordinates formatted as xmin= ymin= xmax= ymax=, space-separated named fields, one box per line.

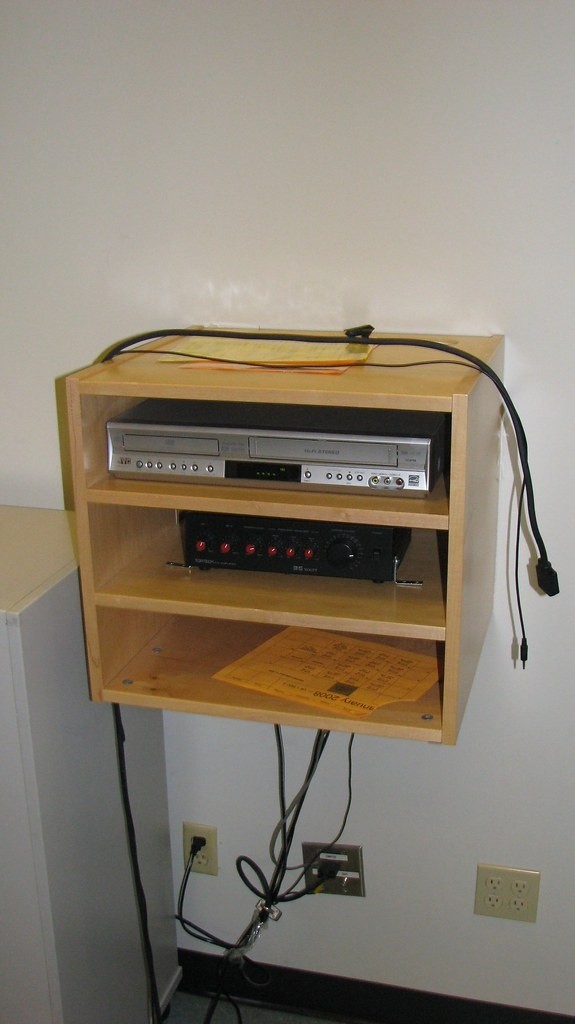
xmin=301 ymin=842 xmax=365 ymax=897
xmin=473 ymin=865 xmax=541 ymax=922
xmin=183 ymin=822 xmax=219 ymax=876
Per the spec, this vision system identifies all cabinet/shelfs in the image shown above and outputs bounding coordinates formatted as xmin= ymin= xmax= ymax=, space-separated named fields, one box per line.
xmin=65 ymin=330 xmax=506 ymax=744
